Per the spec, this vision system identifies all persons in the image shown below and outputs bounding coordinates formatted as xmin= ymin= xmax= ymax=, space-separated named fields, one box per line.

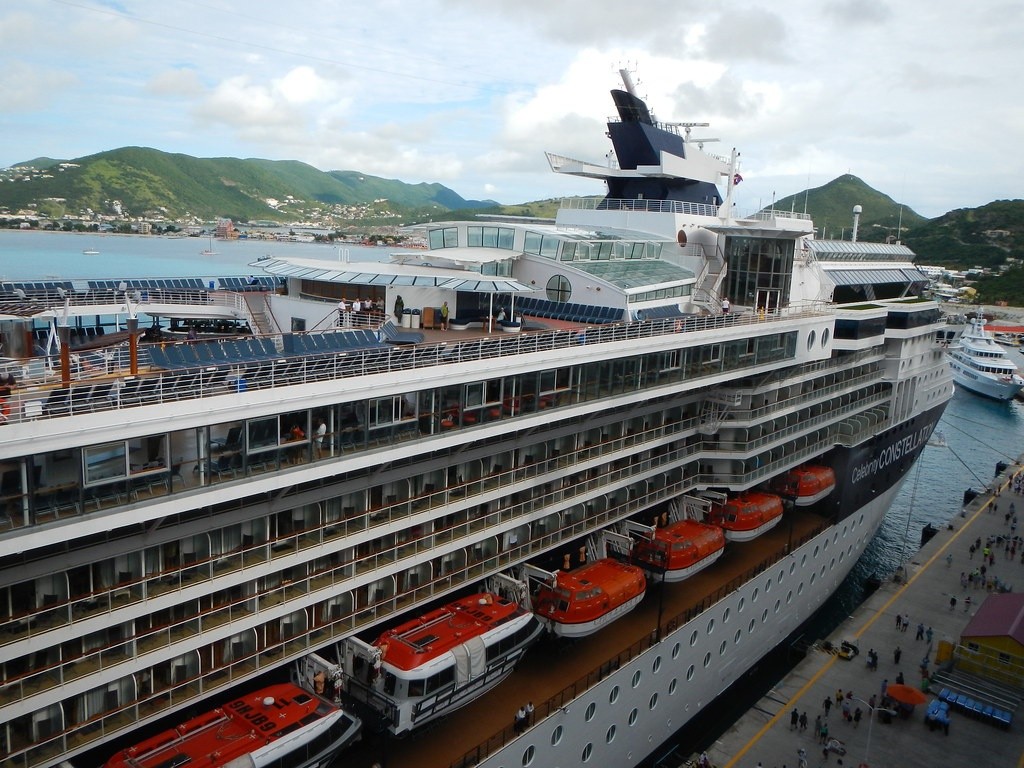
xmin=248 ymin=275 xmax=255 ymax=284
xmin=721 ymin=297 xmax=729 ymax=315
xmin=495 ymin=307 xmax=507 ymax=324
xmin=516 ymin=702 xmax=534 ymax=732
xmin=441 ymin=301 xmax=448 ymax=330
xmin=0 ymin=373 xmax=16 ymax=425
xmin=286 ymin=418 xmax=327 ymax=464
xmin=337 ymin=298 xmax=347 ymax=326
xmin=697 ymin=470 xmax=1024 ymax=768
xmin=187 ymin=329 xmax=197 ymax=341
xmin=352 ymin=296 xmax=385 ymax=327
xmin=632 ymin=309 xmax=642 ymax=322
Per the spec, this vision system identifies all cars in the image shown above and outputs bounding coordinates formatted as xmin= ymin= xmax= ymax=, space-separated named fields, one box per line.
xmin=948 ymin=298 xmax=966 ymax=303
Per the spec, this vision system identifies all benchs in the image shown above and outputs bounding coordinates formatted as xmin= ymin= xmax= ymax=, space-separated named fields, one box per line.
xmin=448 ymin=318 xmax=521 ymax=333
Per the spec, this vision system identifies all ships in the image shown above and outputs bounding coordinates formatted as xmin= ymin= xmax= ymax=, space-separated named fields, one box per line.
xmin=0 ymin=69 xmax=955 ymax=768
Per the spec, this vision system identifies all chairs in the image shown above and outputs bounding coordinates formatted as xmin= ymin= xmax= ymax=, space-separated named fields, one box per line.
xmin=924 ymin=689 xmax=1011 ymax=731
xmin=0 ymin=276 xmax=740 ymax=744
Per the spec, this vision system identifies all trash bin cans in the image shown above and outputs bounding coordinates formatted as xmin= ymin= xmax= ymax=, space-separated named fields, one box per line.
xmin=412 ymin=309 xmax=420 ymax=328
xmin=401 ymin=308 xmax=411 ymax=328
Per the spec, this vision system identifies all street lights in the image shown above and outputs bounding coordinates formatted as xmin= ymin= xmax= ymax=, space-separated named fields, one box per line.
xmin=852 ymin=697 xmax=897 ymax=764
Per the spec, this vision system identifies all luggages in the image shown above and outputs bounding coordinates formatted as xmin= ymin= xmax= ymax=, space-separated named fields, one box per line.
xmin=847 ymin=714 xmax=852 ymax=721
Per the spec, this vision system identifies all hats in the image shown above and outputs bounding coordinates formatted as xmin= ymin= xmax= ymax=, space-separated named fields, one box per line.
xmin=501 ymin=308 xmax=504 ymax=309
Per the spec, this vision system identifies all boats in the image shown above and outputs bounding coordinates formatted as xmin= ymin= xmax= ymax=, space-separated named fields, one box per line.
xmin=101 ymin=683 xmax=364 ymax=768
xmin=632 ymin=518 xmax=725 ymax=582
xmin=531 ymin=558 xmax=647 ymax=638
xmin=935 ymin=315 xmax=967 ymax=353
xmin=707 ymin=491 xmax=783 ymax=542
xmin=944 ymin=307 xmax=1024 ymax=401
xmin=774 ymin=464 xmax=836 ymax=506
xmin=338 ymin=592 xmax=544 ymax=736
